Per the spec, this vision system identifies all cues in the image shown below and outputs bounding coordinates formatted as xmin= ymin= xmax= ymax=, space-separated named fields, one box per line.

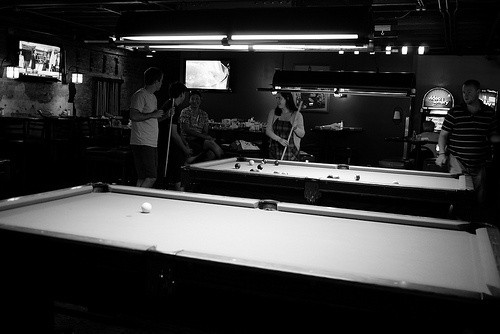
xmin=164 ymin=97 xmax=175 ymax=178
xmin=281 ymin=101 xmax=302 ymax=159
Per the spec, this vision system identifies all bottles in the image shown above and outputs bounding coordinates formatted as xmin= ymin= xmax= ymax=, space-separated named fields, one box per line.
xmin=1 ymin=106 xmax=4 ymax=116
xmin=30 ymin=105 xmax=35 ymax=114
xmin=67 ymin=108 xmax=70 ymax=116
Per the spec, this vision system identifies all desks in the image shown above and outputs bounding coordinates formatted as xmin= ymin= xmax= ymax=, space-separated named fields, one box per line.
xmin=0 ymin=181 xmax=500 ymax=334
xmin=180 ymin=155 xmax=474 ymax=218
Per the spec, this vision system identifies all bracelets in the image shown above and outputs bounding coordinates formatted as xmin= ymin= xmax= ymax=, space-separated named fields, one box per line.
xmin=439 ymin=153 xmax=445 ymax=155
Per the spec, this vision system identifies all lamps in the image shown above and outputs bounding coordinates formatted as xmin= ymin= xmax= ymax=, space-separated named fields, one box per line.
xmin=110 ymin=33 xmax=428 ymax=55
xmin=67 ymin=65 xmax=84 ymax=85
xmin=0 ymin=56 xmax=19 ymax=79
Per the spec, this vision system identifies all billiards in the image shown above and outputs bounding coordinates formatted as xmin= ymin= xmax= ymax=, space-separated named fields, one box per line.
xmin=235 ymin=163 xmax=240 ymax=169
xmin=256 ymin=164 xmax=264 ymax=170
xmin=274 ymin=161 xmax=279 ymax=166
xmin=392 ymin=181 xmax=399 ymax=184
xmin=334 ymin=177 xmax=339 ymax=179
xmin=249 ymin=160 xmax=254 ymax=166
xmin=142 ymin=201 xmax=152 ymax=212
xmin=356 ymin=175 xmax=360 ymax=180
xmin=282 ymin=172 xmax=288 ymax=175
xmin=327 ymin=175 xmax=333 ymax=178
xmin=273 ymin=171 xmax=279 ymax=174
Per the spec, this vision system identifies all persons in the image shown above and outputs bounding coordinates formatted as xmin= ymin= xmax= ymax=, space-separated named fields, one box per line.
xmin=128 ymin=65 xmax=166 ymax=191
xmin=177 ymin=91 xmax=226 ymax=166
xmin=158 ymin=81 xmax=194 ymax=192
xmin=432 ymin=80 xmax=500 ymax=223
xmin=264 ymin=91 xmax=305 ymax=167
xmin=18 ymin=43 xmax=60 ymax=76
